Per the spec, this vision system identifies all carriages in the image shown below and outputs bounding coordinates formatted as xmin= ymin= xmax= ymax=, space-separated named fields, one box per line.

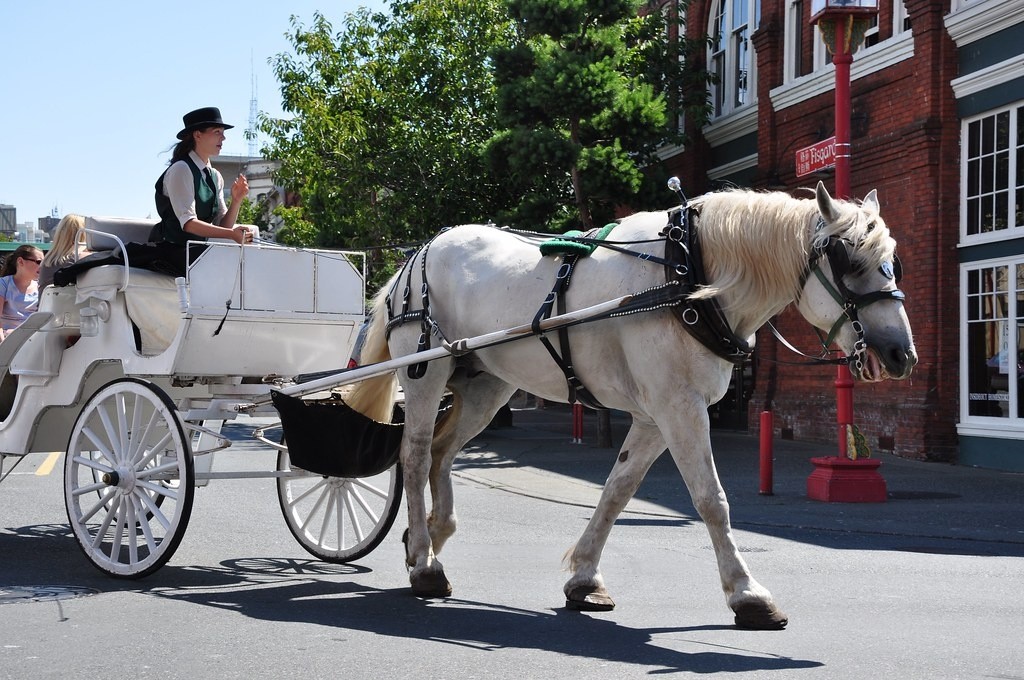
xmin=0 ymin=176 xmax=919 ymax=633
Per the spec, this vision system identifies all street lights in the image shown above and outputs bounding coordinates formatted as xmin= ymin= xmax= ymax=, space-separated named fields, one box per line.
xmin=804 ymin=0 xmax=889 ymax=502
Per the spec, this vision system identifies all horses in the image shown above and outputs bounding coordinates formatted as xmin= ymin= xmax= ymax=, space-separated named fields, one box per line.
xmin=337 ymin=179 xmax=917 ymax=629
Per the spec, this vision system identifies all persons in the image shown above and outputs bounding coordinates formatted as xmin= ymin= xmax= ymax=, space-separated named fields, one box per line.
xmin=0 ymin=245 xmax=44 ymax=345
xmin=148 ymin=108 xmax=253 ymax=279
xmin=37 ymin=214 xmax=91 ymax=311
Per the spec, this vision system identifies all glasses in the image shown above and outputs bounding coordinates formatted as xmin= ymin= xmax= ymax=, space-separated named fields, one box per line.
xmin=23 ymin=258 xmax=42 ymax=265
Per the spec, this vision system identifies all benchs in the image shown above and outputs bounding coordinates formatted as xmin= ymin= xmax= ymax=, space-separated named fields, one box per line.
xmin=38 ymin=282 xmax=82 ymax=329
xmin=72 ymin=216 xmax=259 ymax=292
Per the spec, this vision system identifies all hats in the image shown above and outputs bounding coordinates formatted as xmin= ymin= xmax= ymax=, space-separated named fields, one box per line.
xmin=177 ymin=107 xmax=234 ymax=140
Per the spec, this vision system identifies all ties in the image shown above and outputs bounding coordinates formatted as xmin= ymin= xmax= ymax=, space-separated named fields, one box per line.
xmin=203 ymin=167 xmax=216 ymax=197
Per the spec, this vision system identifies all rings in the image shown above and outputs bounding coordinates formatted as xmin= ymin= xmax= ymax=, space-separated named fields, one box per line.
xmin=246 ymin=239 xmax=248 ymax=241
